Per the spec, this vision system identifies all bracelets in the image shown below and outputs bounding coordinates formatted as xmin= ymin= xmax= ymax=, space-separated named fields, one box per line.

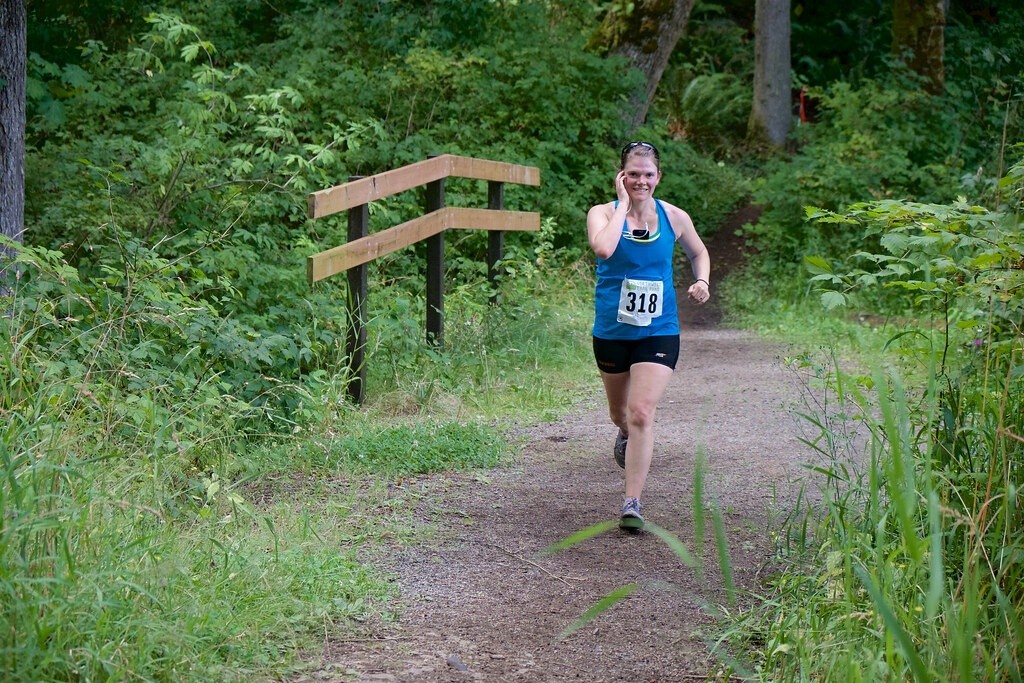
xmin=695 ymin=279 xmax=710 ymax=287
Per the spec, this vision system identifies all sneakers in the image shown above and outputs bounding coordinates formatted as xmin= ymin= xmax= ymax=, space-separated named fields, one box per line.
xmin=614 ymin=430 xmax=628 ymax=468
xmin=618 ymin=498 xmax=643 ymax=535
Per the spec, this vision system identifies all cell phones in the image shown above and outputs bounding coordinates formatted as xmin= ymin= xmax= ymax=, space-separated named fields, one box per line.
xmin=633 ymin=230 xmax=649 ymax=240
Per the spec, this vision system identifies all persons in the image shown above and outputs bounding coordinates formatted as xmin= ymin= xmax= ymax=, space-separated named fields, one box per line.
xmin=586 ymin=141 xmax=710 ymax=530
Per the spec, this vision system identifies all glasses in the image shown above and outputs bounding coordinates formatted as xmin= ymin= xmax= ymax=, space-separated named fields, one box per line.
xmin=621 ymin=141 xmax=658 ymax=162
